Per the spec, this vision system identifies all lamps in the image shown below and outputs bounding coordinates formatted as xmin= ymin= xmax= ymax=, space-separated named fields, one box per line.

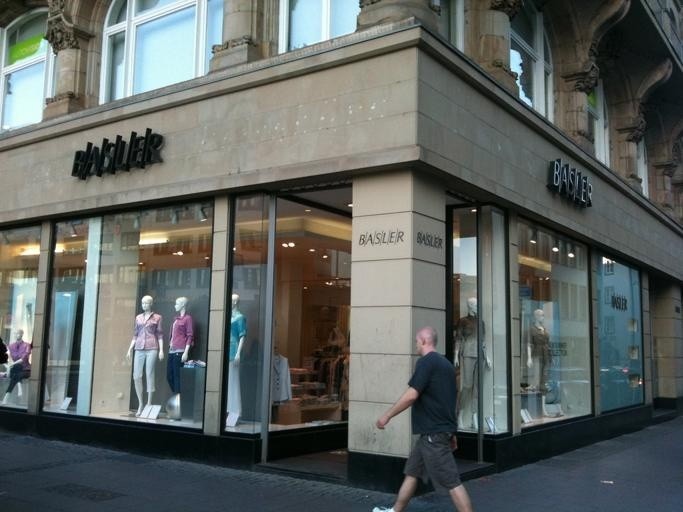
xmin=134 ymin=212 xmax=149 ymax=229
xmin=517 ymin=218 xmax=584 ymax=259
xmin=70 ymin=220 xmax=78 ymax=237
xmin=3 ymin=232 xmax=9 ymax=244
xmin=200 ymin=201 xmax=213 ymax=222
xmin=171 ymin=208 xmax=182 ymax=224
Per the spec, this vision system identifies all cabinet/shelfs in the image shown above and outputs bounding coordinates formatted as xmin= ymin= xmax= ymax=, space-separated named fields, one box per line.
xmin=273 ymin=368 xmax=342 ymax=425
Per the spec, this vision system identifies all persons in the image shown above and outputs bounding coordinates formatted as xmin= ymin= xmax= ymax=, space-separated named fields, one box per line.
xmin=165 ymin=296 xmax=194 ymax=395
xmin=451 ymin=296 xmax=493 ymax=430
xmin=124 ymin=293 xmax=165 ymax=416
xmin=226 ymin=293 xmax=246 ymax=417
xmin=1 ymin=327 xmax=33 ymax=405
xmin=525 ymin=307 xmax=552 ymax=416
xmin=374 ymin=325 xmax=474 ymax=512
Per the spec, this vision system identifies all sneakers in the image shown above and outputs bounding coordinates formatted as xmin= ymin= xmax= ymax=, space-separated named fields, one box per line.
xmin=372 ymin=507 xmax=393 ymax=512
